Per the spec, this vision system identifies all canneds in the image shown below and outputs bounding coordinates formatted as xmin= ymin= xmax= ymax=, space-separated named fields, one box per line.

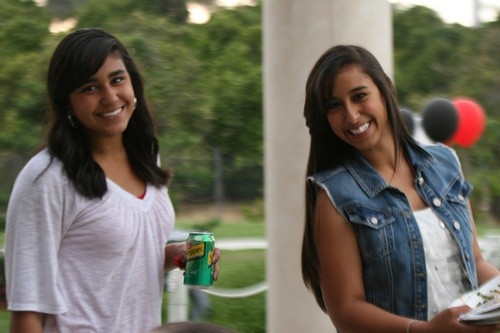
xmin=184 ymin=232 xmax=216 ymax=290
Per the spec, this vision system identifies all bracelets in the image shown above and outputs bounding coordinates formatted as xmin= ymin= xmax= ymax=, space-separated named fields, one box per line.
xmin=407 ymin=318 xmax=416 ymax=333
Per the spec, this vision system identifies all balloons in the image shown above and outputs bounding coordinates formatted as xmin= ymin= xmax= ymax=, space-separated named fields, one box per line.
xmin=399 ymin=96 xmax=485 ymax=148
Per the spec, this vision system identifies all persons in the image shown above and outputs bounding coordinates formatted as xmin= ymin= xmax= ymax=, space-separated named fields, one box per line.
xmin=3 ymin=28 xmax=220 ymax=333
xmin=301 ymin=45 xmax=499 ymax=333
